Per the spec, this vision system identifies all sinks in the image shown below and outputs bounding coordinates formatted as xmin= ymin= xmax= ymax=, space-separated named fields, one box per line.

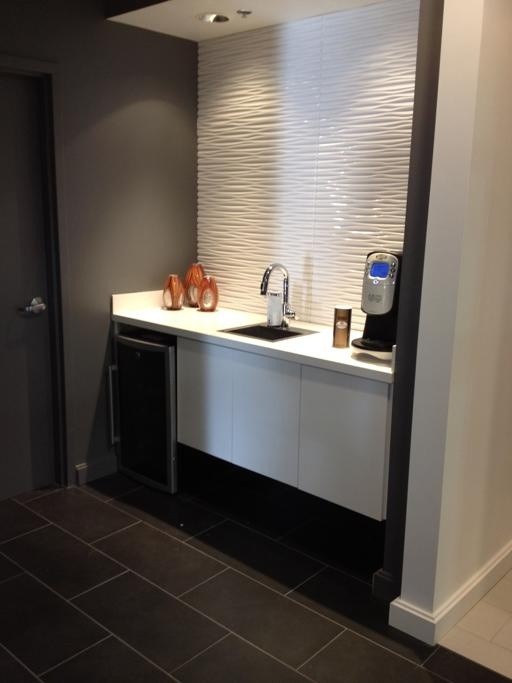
xmin=217 ymin=322 xmax=321 ymax=343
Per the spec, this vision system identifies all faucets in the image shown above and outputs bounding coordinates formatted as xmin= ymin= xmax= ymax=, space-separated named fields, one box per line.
xmin=260 ymin=263 xmax=295 ymax=328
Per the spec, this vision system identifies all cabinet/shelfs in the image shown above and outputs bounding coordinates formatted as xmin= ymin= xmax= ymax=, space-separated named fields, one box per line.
xmin=114 ymin=322 xmax=175 ymax=495
xmin=177 ymin=337 xmax=393 ymax=521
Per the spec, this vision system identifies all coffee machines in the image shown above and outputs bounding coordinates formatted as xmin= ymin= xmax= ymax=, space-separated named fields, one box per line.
xmin=351 ymin=249 xmax=403 ymax=352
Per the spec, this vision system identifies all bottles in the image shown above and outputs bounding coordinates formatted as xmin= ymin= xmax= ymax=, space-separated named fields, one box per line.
xmin=267 ymin=290 xmax=282 ymax=326
xmin=333 ymin=305 xmax=352 ymax=348
